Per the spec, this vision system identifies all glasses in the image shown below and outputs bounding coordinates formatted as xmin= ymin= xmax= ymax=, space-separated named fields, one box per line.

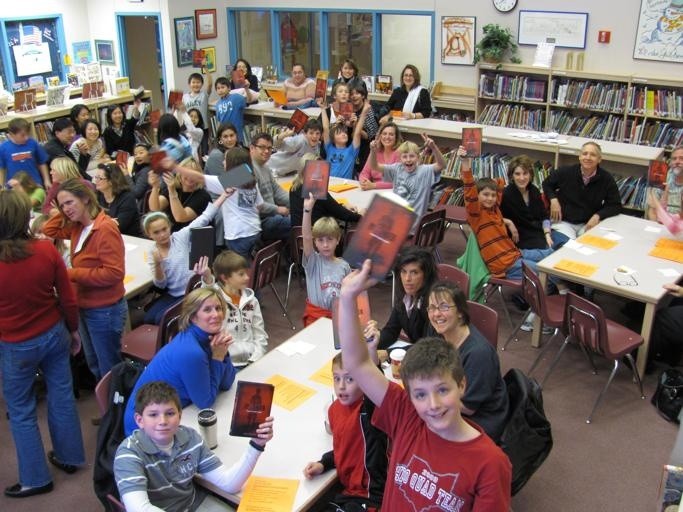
xmin=426 ymin=304 xmax=458 ymax=312
xmin=255 ymin=144 xmax=275 ymax=153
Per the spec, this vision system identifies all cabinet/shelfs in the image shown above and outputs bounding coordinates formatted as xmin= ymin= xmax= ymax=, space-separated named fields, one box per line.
xmin=1 ymin=89 xmax=155 ymax=145
xmin=430 ymin=59 xmax=683 ymax=152
xmin=191 ymin=95 xmax=328 ymax=154
xmin=376 ymin=113 xmax=663 ymax=219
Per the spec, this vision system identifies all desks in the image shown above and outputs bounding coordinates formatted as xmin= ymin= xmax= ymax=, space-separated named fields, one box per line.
xmin=272 ymin=172 xmax=411 ymax=222
xmin=531 ymin=213 xmax=683 ymax=384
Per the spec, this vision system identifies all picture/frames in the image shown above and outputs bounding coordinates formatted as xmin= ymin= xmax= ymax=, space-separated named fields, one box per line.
xmin=175 ymin=15 xmax=196 ymax=67
xmin=442 ymin=16 xmax=476 ymax=67
xmin=196 ymin=9 xmax=218 ymax=40
xmin=632 ymin=0 xmax=683 ymax=61
xmin=201 ymin=48 xmax=217 ymax=74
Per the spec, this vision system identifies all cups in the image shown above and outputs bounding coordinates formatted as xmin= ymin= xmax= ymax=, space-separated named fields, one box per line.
xmin=388 ymin=347 xmax=409 ymax=378
xmin=198 ymin=408 xmax=220 ymax=450
xmin=615 ymin=265 xmax=632 ymax=280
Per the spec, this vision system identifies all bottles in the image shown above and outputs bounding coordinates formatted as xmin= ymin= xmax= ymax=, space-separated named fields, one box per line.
xmin=264 ymin=64 xmax=278 ymax=84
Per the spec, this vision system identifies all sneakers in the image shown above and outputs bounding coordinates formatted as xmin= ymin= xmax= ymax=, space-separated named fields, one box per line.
xmin=519 ymin=320 xmax=555 ymax=335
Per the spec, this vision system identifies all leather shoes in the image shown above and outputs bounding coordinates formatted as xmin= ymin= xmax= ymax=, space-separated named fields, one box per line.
xmin=3 ymin=479 xmax=54 ymax=498
xmin=47 ymin=450 xmax=77 ymax=474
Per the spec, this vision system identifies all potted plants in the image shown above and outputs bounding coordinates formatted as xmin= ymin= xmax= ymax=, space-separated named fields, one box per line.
xmin=477 ymin=24 xmax=521 ymax=72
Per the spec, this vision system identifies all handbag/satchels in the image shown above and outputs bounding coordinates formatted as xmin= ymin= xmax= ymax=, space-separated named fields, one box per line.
xmin=650 ymin=368 xmax=683 ymax=423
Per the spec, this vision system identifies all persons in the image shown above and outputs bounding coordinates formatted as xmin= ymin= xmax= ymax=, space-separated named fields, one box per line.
xmin=622 ymin=148 xmax=682 ymax=375
xmin=458 ymin=145 xmax=573 ymax=296
xmin=543 ymin=142 xmax=623 ymax=239
xmin=0 ymin=57 xmax=510 ymax=512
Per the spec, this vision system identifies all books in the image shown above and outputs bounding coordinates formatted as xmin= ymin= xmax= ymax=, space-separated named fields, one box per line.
xmin=477 ymin=71 xmax=682 ymax=149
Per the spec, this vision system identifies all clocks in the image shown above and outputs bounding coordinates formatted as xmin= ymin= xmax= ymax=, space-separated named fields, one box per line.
xmin=490 ymin=0 xmax=519 ymax=13
